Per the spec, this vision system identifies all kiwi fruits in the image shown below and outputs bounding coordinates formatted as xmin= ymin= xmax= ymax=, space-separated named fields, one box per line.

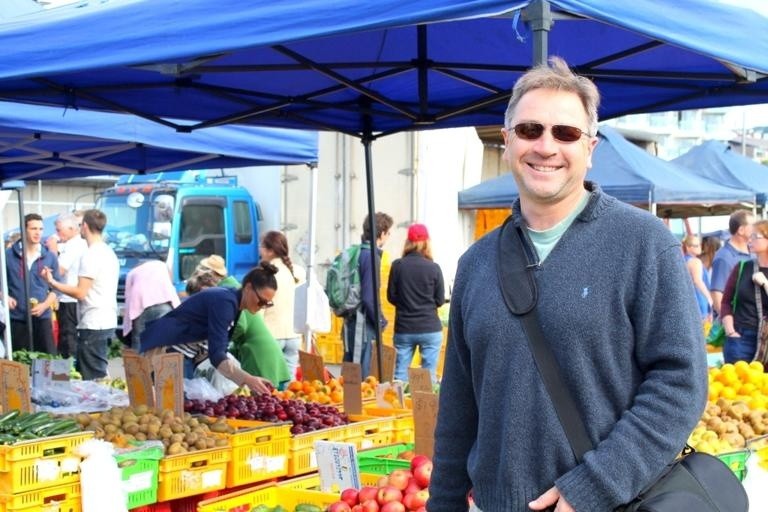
xmin=692 ymin=398 xmax=768 ymax=448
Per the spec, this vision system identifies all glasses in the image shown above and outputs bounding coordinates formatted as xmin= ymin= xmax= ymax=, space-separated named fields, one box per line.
xmin=507 ymin=121 xmax=593 ymax=144
xmin=253 ymin=289 xmax=274 ymax=310
xmin=749 ymin=232 xmax=765 ymax=240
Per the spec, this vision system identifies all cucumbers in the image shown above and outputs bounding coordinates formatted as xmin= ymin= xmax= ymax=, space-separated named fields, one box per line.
xmin=0 ymin=409 xmax=79 ymax=455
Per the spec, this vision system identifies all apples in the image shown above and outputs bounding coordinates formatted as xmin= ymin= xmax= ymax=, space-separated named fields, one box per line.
xmin=328 ymin=455 xmax=432 ymax=512
xmin=265 ymin=383 xmax=275 ymax=391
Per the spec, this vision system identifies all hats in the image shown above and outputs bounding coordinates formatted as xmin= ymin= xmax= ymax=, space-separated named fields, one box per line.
xmin=408 ymin=224 xmax=430 ymax=242
xmin=200 ymin=254 xmax=228 ymax=277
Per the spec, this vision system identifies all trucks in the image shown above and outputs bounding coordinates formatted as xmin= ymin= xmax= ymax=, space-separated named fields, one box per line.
xmin=68 ymin=124 xmax=485 ymax=346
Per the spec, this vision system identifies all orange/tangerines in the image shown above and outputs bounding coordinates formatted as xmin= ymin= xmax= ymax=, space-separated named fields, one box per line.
xmin=273 ymin=376 xmax=376 ymax=404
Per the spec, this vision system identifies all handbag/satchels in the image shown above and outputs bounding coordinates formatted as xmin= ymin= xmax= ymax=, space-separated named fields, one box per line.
xmin=753 ymin=318 xmax=768 ymax=368
xmin=707 ymin=313 xmax=730 ymax=347
xmin=620 ymin=446 xmax=754 ymax=512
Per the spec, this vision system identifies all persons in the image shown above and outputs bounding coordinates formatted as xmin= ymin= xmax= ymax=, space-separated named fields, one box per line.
xmin=423 ymin=52 xmax=712 ymax=512
xmin=386 ymin=221 xmax=446 ymax=391
xmin=139 ymin=259 xmax=279 ymax=401
xmin=680 ymin=208 xmax=768 ymax=375
xmin=0 ymin=207 xmax=125 ymax=380
xmin=338 ymin=210 xmax=396 ymax=380
xmin=185 ymin=269 xmax=293 ymax=392
xmin=121 ymin=259 xmax=183 ymax=350
xmin=199 ymin=253 xmax=228 ymax=282
xmin=255 ymin=229 xmax=302 ymax=391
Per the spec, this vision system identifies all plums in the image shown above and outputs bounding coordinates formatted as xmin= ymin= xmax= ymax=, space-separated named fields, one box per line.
xmin=57 ymin=394 xmax=348 ymax=455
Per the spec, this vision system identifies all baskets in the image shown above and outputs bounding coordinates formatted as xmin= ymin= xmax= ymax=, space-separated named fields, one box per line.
xmin=200 ymin=414 xmax=293 ymax=490
xmin=289 ymin=395 xmax=418 ymax=479
xmin=0 ymin=431 xmax=94 ymax=512
xmin=114 ymin=441 xmax=164 ymax=509
xmin=159 ymin=441 xmax=232 ymax=503
xmin=276 ymin=469 xmax=384 ymax=499
xmin=355 ymin=442 xmax=418 ymax=477
xmin=197 ymin=481 xmax=341 ymax=512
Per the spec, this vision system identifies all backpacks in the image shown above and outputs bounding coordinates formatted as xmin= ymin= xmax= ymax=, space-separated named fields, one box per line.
xmin=326 ymin=244 xmax=373 ymax=319
